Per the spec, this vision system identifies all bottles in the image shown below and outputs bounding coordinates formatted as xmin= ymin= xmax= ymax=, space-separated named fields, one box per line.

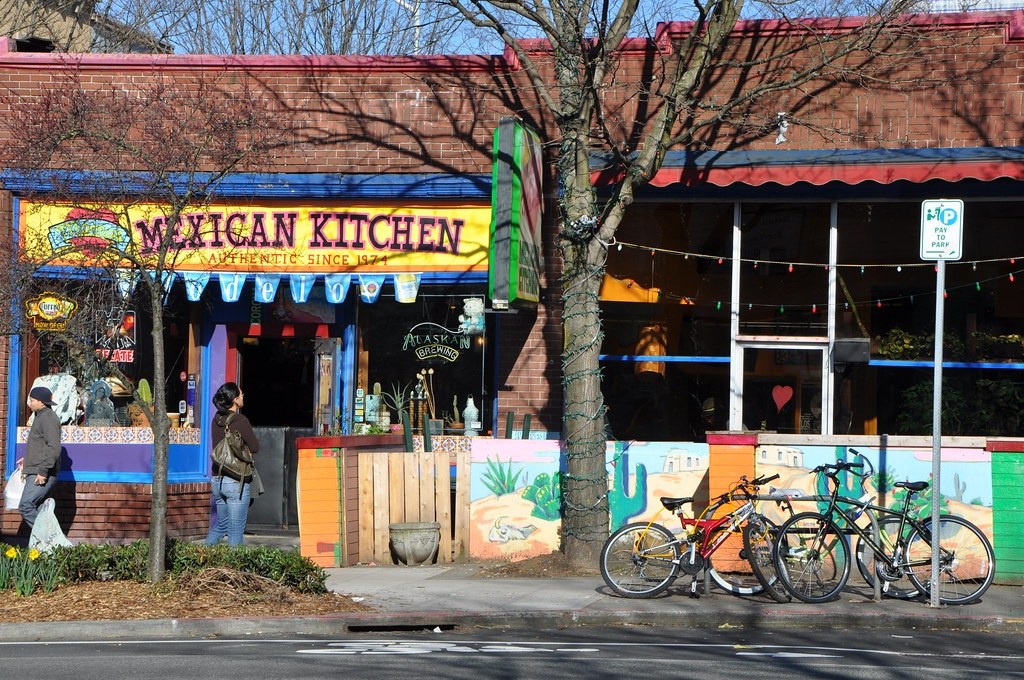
xmin=398 ymin=274 xmax=417 ymax=303
xmin=462 ymin=397 xmax=479 ymax=436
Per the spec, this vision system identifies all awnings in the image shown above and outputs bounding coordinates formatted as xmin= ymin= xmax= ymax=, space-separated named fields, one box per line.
xmin=20 ymin=201 xmax=493 ymax=282
xmin=556 ymin=159 xmax=1024 ymax=188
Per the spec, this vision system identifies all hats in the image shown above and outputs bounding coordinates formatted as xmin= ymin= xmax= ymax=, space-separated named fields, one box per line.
xmin=30 ymin=387 xmax=58 ymax=406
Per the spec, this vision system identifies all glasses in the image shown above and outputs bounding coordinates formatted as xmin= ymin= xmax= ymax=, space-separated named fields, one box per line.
xmin=234 ymin=392 xmax=244 ymax=399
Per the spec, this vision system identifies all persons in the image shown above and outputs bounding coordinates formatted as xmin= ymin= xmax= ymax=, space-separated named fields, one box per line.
xmin=18 ymin=387 xmax=61 ymax=526
xmin=204 ymin=381 xmax=259 ymax=547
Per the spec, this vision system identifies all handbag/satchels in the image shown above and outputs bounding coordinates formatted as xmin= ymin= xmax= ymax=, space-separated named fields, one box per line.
xmin=4 ymin=465 xmax=26 ymax=511
xmin=211 ymin=413 xmax=254 ymax=478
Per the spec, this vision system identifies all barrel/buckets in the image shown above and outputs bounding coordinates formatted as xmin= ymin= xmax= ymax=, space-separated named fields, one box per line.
xmin=167 ymin=413 xmax=181 ymax=428
xmin=115 ymin=406 xmax=133 ymax=427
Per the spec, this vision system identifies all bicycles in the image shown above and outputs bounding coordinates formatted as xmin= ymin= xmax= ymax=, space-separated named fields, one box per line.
xmin=709 ymin=448 xmax=942 ymax=600
xmin=599 ymin=473 xmax=793 ymax=604
xmin=772 ymin=462 xmax=996 ymax=604
